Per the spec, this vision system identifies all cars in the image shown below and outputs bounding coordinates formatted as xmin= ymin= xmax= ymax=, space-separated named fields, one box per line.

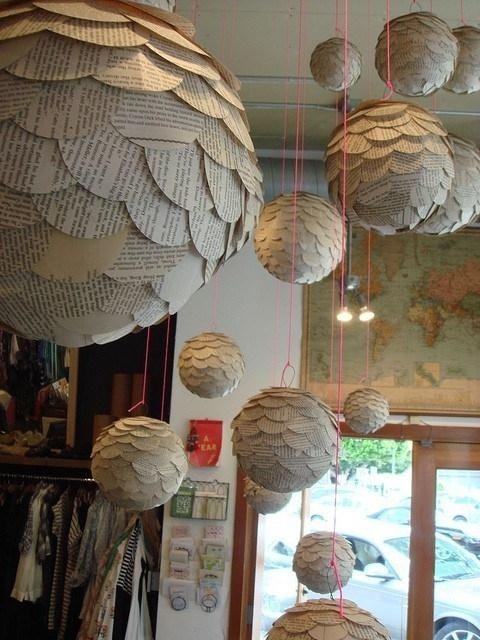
xmin=260 ymin=494 xmax=480 ymax=639
